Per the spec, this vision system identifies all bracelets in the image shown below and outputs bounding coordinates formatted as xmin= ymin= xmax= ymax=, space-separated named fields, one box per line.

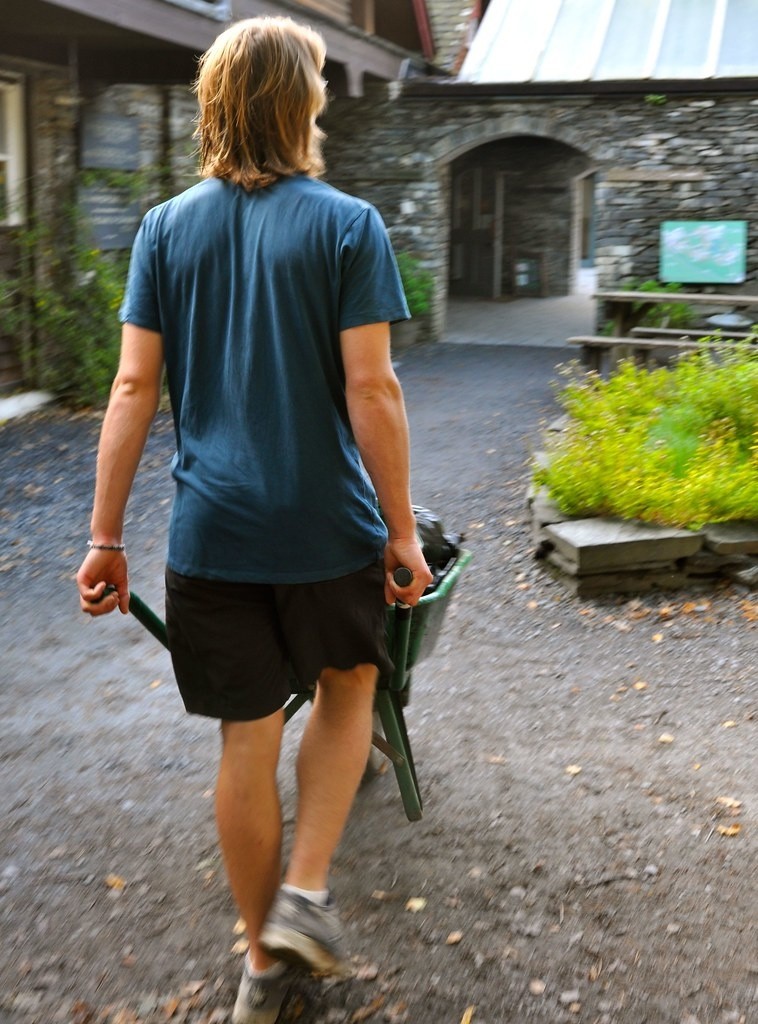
xmin=87 ymin=542 xmax=125 ymax=550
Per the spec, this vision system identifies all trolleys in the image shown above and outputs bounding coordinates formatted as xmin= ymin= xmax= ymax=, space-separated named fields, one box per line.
xmin=88 ymin=504 xmax=474 ymax=823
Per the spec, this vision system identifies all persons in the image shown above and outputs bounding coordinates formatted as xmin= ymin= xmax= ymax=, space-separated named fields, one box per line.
xmin=78 ymin=18 xmax=433 ymax=1024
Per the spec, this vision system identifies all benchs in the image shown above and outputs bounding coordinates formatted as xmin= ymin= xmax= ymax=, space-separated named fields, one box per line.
xmin=565 ymin=335 xmax=758 ymax=375
xmin=631 ymin=326 xmax=758 ymax=342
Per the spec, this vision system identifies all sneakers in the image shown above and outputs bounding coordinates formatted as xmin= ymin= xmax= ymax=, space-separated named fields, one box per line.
xmin=230 ymin=951 xmax=294 ymax=1024
xmin=258 ymin=887 xmax=354 ymax=976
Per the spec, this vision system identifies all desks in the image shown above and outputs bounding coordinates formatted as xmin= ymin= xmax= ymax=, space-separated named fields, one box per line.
xmin=589 ymin=291 xmax=758 ymax=391
